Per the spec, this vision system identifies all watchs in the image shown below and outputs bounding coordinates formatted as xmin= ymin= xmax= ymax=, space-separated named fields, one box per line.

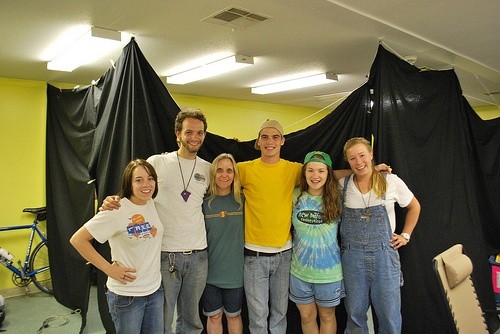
xmin=400 ymin=233 xmax=410 ymax=240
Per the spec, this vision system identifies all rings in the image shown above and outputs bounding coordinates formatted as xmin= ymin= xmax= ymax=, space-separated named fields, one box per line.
xmin=123 ymin=276 xmax=125 ymax=279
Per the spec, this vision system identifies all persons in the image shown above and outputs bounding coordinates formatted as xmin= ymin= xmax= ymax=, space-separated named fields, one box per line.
xmin=339 ymin=137 xmax=422 ymax=334
xmin=70 ymin=159 xmax=165 ymax=334
xmin=236 ymin=120 xmax=391 ymax=334
xmin=290 ymin=151 xmax=347 ymax=334
xmin=203 ymin=153 xmax=245 ymax=334
xmin=98 ymin=110 xmax=212 ymax=333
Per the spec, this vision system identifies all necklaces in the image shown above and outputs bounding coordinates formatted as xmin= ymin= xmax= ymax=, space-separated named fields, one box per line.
xmin=355 ymin=177 xmax=372 ymax=220
xmin=177 ymin=153 xmax=196 ymax=202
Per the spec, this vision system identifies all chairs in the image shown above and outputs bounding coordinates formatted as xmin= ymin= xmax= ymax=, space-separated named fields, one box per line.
xmin=433 ymin=244 xmax=490 ymax=334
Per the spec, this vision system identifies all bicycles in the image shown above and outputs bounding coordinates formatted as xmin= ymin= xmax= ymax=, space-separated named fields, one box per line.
xmin=0 ymin=206 xmax=52 ymax=295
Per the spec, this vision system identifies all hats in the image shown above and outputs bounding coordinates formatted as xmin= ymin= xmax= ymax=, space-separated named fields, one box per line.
xmin=255 ymin=119 xmax=282 ymax=150
xmin=304 ymin=152 xmax=333 ymax=167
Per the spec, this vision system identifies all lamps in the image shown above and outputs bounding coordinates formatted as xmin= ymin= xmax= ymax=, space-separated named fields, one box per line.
xmin=251 ymin=72 xmax=339 ymax=94
xmin=46 ymin=27 xmax=122 ymax=73
xmin=166 ymin=55 xmax=254 ymax=85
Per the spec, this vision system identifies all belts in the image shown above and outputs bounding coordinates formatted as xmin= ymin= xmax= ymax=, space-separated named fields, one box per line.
xmin=244 ymin=248 xmax=291 ymax=256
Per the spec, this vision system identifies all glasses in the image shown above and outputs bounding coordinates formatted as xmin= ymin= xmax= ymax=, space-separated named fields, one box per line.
xmin=216 ymin=168 xmax=233 ymax=173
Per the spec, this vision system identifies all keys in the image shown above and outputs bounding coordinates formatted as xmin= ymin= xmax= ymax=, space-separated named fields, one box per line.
xmin=169 ymin=267 xmax=176 ymax=277
